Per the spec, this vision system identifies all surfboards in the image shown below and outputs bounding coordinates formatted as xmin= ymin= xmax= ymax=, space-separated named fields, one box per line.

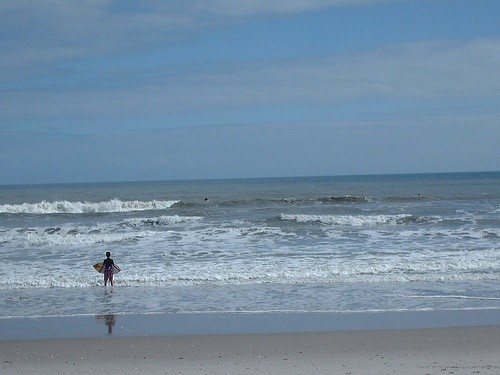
xmin=92 ymin=262 xmax=121 ymax=274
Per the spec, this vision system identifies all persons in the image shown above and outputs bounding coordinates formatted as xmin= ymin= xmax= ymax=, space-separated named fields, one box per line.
xmin=99 ymin=251 xmax=121 ymax=286
